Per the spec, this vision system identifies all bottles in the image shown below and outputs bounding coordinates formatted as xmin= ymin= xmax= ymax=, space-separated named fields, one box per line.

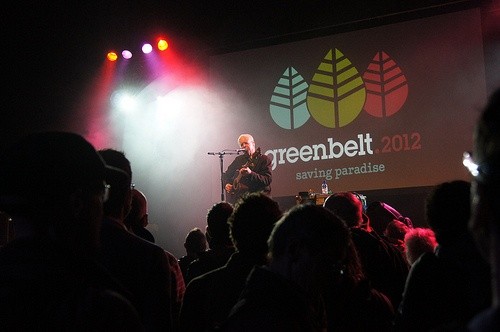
xmin=322 ymin=180 xmax=328 ymax=194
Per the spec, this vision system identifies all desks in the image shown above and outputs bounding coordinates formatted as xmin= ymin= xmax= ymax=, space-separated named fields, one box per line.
xmin=295 ymin=193 xmax=330 ymax=205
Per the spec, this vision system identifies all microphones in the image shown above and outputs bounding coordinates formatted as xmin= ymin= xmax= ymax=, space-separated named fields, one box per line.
xmin=235 ymin=148 xmax=247 ymax=151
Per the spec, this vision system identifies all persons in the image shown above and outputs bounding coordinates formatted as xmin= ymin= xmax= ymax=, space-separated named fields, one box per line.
xmin=222 ymin=134 xmax=272 ymax=198
xmin=178 ymin=88 xmax=500 ymax=332
xmin=0 ymin=131 xmax=185 ymax=332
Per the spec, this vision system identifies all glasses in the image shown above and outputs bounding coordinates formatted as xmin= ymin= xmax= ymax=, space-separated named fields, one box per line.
xmin=82 ymin=184 xmax=111 ymax=201
xmin=130 ymin=184 xmax=135 ymax=191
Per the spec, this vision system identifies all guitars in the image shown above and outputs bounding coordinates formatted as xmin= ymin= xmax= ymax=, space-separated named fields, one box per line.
xmin=226 ymin=146 xmax=262 ymax=208
